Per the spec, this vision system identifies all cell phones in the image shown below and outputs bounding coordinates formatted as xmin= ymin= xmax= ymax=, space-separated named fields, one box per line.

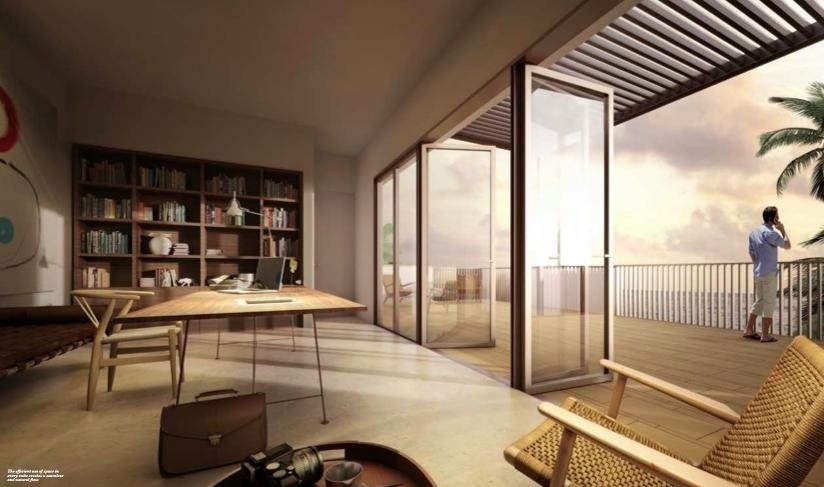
xmin=773 ymin=217 xmax=778 ymax=224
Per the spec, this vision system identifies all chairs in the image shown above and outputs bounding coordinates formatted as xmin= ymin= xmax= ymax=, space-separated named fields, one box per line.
xmin=382 ymin=272 xmax=414 ymax=309
xmin=503 ymin=333 xmax=824 ymax=486
xmin=68 ymin=285 xmax=189 ymax=414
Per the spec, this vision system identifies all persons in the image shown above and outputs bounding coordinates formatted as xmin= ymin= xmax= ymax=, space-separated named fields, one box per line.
xmin=741 ymin=205 xmax=792 ymax=341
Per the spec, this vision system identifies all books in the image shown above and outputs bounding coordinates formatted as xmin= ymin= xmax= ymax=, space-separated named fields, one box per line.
xmin=79 ymin=154 xmax=304 ymax=290
xmin=217 ymin=288 xmax=278 ymax=295
xmin=246 ymin=294 xmax=292 ymax=303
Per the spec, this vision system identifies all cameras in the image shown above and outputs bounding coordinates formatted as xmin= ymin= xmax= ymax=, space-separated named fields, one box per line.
xmin=240 ymin=442 xmax=324 ymax=487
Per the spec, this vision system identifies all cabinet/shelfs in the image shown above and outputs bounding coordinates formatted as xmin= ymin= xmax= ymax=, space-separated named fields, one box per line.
xmin=68 ymin=139 xmax=306 ymax=331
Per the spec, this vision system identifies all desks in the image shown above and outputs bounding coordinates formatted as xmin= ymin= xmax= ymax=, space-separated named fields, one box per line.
xmin=211 ymin=437 xmax=439 ymax=486
xmin=114 ymin=283 xmax=368 ymax=427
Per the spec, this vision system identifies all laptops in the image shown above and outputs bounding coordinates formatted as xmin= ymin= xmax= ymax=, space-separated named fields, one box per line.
xmin=218 ymin=256 xmax=287 ymax=294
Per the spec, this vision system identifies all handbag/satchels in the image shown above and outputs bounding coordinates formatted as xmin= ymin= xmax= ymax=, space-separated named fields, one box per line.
xmin=158 ymin=389 xmax=268 ymax=476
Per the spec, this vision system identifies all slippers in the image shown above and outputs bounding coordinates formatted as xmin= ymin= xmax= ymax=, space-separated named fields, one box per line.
xmin=742 ymin=332 xmax=778 ymax=342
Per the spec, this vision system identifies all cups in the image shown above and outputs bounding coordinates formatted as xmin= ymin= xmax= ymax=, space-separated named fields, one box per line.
xmin=324 ymin=461 xmax=363 ymax=487
